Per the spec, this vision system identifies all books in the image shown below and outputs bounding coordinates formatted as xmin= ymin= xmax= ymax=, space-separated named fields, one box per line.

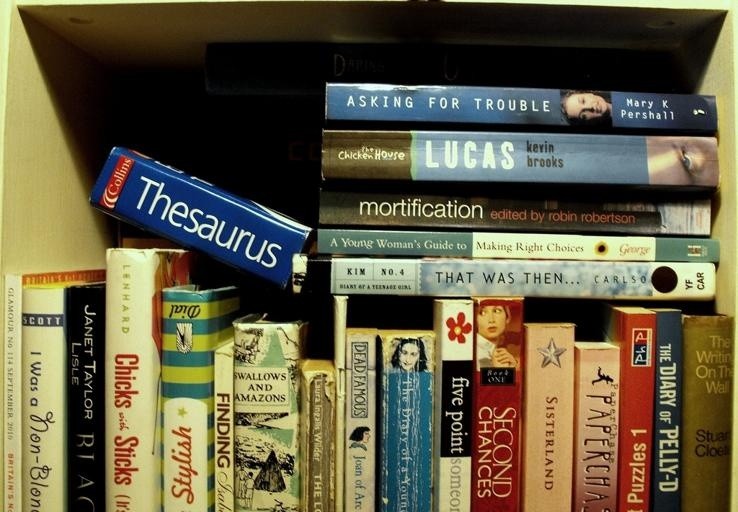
xmin=3 ymin=83 xmax=737 ymax=512
